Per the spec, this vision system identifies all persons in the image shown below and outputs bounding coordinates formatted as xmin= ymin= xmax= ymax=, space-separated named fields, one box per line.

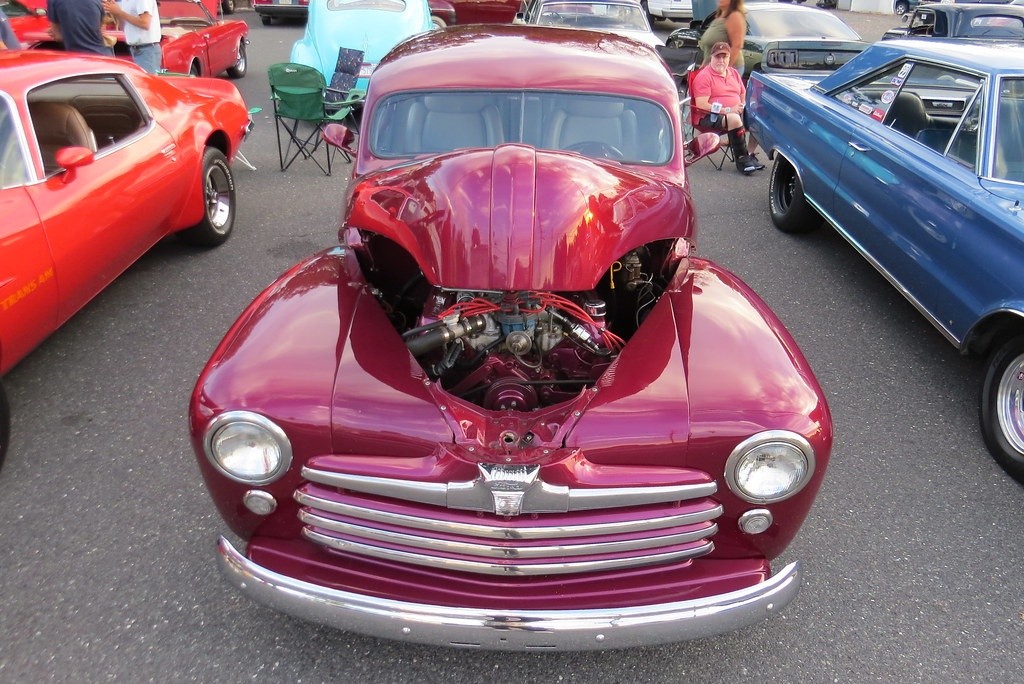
xmin=104 ymin=0 xmax=163 ymax=71
xmin=696 ymin=0 xmax=746 ymax=75
xmin=47 ymin=0 xmax=113 ymax=56
xmin=0 ymin=9 xmax=21 ymax=50
xmin=692 ymin=41 xmax=766 ymax=174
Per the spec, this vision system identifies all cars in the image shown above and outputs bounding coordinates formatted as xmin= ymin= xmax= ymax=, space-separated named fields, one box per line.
xmin=0 ymin=0 xmax=309 ymax=81
xmin=743 ymin=31 xmax=1024 ymax=484
xmin=0 ymin=48 xmax=255 ymax=461
xmin=882 ymin=0 xmax=1024 ymax=42
xmin=639 ymin=0 xmax=873 ymax=76
xmin=289 ymin=0 xmax=510 ymax=109
xmin=189 ymin=17 xmax=834 ymax=653
xmin=512 ymin=0 xmax=667 ymax=49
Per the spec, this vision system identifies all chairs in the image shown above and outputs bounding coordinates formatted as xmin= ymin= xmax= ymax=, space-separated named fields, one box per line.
xmin=27 ymin=102 xmax=100 ymax=154
xmin=267 ymin=63 xmax=366 ymax=176
xmin=322 ymin=47 xmax=366 ymax=135
xmin=883 ymin=91 xmax=937 ymax=150
xmin=678 ymin=68 xmax=736 ymax=170
xmin=542 ymin=93 xmax=639 ymax=162
xmin=404 ymin=93 xmax=504 ymax=156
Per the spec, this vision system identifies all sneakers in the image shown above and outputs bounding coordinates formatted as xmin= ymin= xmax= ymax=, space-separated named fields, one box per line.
xmin=735 ymin=155 xmax=755 ymax=172
xmin=749 ymin=152 xmax=765 ymax=169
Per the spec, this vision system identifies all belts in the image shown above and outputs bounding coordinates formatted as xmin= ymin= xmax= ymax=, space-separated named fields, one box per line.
xmin=132 ymin=42 xmax=157 ymax=48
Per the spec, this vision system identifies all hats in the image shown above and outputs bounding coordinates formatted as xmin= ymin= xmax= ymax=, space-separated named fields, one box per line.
xmin=711 ymin=42 xmax=731 ymax=56
